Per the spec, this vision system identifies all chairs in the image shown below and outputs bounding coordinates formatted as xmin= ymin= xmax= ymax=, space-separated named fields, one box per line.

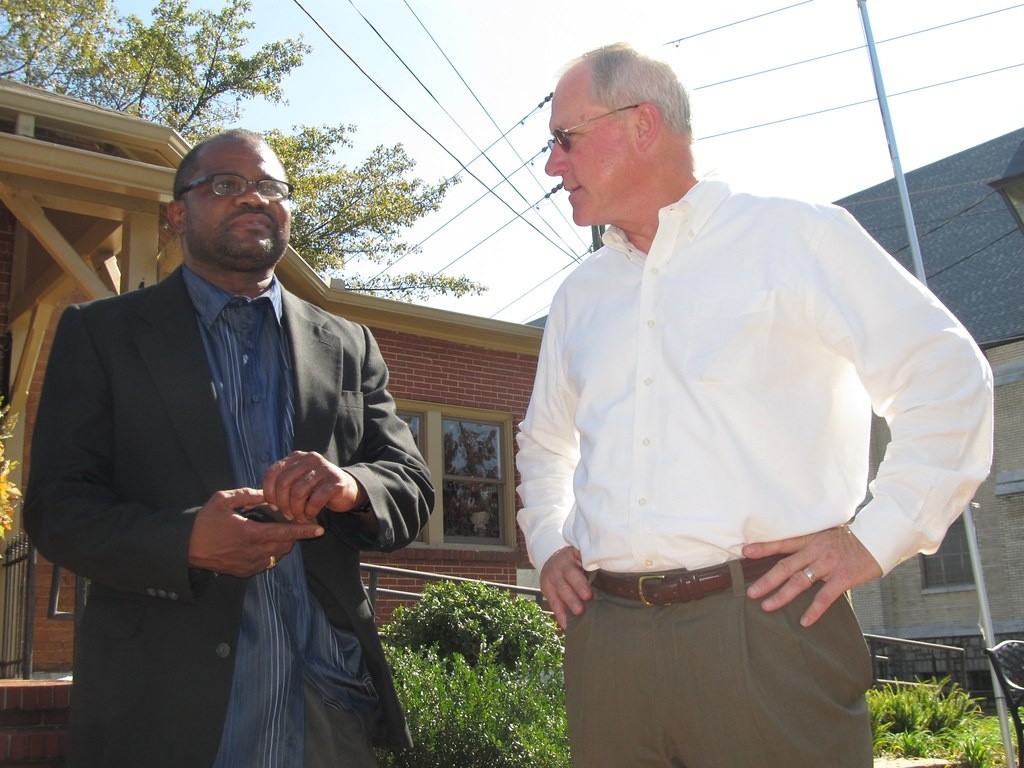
xmin=984 ymin=640 xmax=1024 ymax=768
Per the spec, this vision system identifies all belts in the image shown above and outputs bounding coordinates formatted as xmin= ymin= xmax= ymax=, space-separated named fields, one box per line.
xmin=591 ymin=554 xmax=792 ymax=608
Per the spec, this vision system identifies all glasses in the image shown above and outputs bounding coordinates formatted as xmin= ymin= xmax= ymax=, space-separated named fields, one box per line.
xmin=548 ymin=104 xmax=639 ymax=153
xmin=177 ymin=173 xmax=294 ymax=202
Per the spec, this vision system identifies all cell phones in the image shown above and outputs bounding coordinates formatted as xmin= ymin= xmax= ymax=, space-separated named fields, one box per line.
xmin=240 ymin=503 xmax=288 ymax=523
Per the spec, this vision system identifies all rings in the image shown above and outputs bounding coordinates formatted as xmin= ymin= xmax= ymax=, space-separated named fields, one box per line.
xmin=803 ymin=568 xmax=818 ymax=586
xmin=266 ymin=556 xmax=278 ymax=570
xmin=305 ymin=469 xmax=320 ymax=484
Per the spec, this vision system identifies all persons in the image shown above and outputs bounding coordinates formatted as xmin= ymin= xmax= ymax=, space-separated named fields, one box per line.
xmin=515 ymin=46 xmax=996 ymax=768
xmin=17 ymin=127 xmax=417 ymax=767
